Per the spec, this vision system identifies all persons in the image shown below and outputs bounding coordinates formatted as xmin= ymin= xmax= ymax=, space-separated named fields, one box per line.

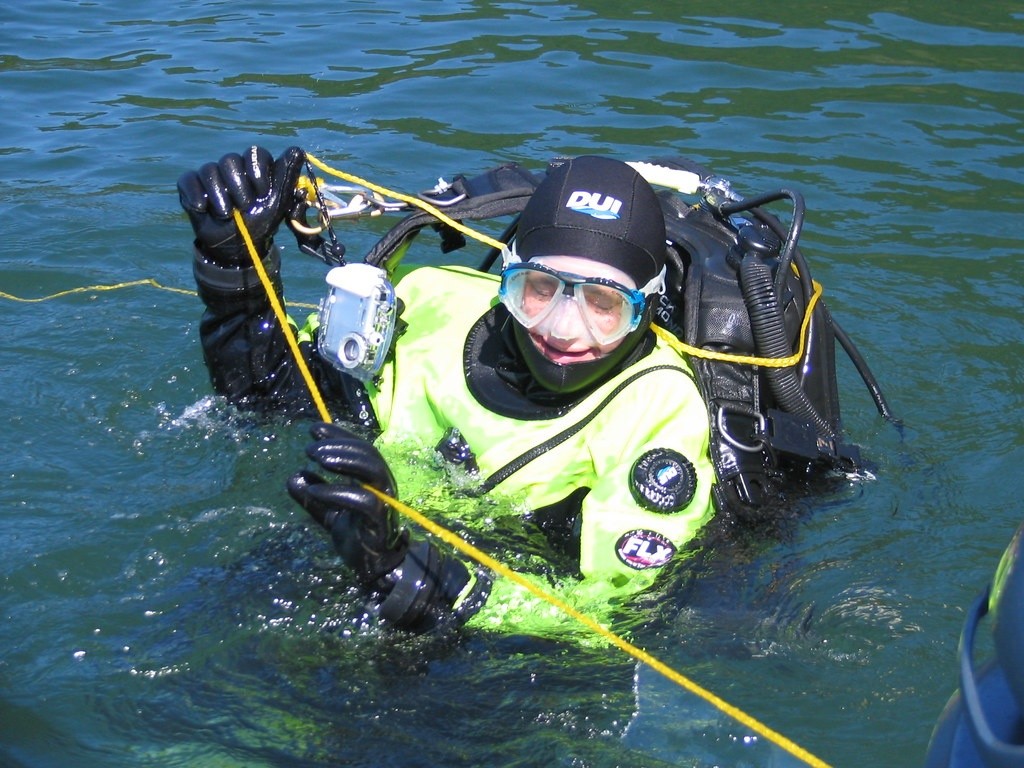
xmin=177 ymin=146 xmax=713 ymax=631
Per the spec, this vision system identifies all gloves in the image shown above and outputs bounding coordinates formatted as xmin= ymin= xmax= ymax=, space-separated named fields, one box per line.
xmin=286 ymin=422 xmax=407 ymax=584
xmin=176 ymin=144 xmax=306 ymax=270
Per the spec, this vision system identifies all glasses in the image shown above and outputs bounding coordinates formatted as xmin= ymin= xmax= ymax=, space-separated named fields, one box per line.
xmin=498 ymin=261 xmax=646 ymax=346
